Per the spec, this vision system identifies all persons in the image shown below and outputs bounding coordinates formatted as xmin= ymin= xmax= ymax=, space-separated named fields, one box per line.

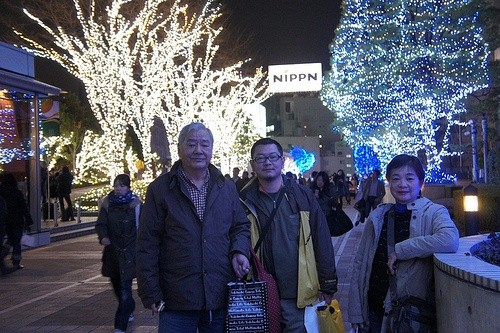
xmin=95 ymin=174 xmax=141 ymax=333
xmin=238 ymin=138 xmax=338 ymax=333
xmin=285 ymin=169 xmax=386 ymax=225
xmin=0 ymin=173 xmax=33 ymax=268
xmin=349 ymin=154 xmax=459 ymax=333
xmin=40 ymin=161 xmax=75 ymax=221
xmin=135 ymin=123 xmax=251 ymax=333
xmin=225 ymin=167 xmax=255 ymax=191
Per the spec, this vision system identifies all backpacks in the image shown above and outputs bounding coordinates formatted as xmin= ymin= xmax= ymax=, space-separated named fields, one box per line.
xmin=64 ymin=206 xmax=75 ymax=221
xmin=43 ymin=202 xmax=55 ymax=221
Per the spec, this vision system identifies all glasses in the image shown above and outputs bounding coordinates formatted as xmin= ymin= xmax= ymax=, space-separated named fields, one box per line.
xmin=251 ymin=152 xmax=283 ymax=163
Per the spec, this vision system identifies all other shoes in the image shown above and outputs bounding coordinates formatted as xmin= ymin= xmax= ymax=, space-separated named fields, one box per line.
xmin=112 ymin=329 xmax=126 ymax=333
xmin=128 ymin=312 xmax=135 ymax=321
xmin=14 ymin=265 xmax=24 ymax=269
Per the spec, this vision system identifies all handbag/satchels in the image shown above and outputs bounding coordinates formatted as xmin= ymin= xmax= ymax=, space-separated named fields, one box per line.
xmin=353 ymin=198 xmax=366 ymax=215
xmin=227 ymin=245 xmax=281 ymax=333
xmin=303 ymin=299 xmax=346 ymax=333
xmin=325 ymin=200 xmax=354 ymax=236
xmin=386 ymin=295 xmax=437 ymax=333
